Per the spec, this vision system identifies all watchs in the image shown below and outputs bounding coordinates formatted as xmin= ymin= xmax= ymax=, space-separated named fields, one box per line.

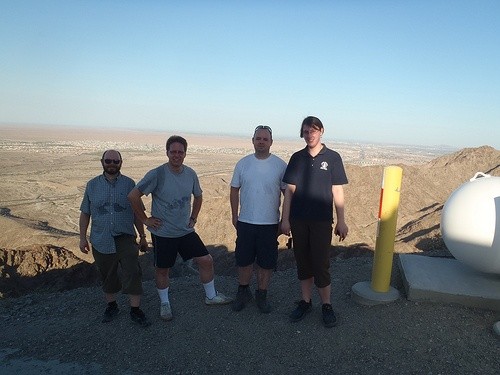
xmin=140 ymin=234 xmax=146 ymax=237
xmin=190 ymin=217 xmax=197 ymax=223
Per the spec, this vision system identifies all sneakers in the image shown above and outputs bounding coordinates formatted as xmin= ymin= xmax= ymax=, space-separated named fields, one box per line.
xmin=160 ymin=299 xmax=173 ymax=322
xmin=288 ymin=298 xmax=313 ymax=323
xmin=205 ymin=291 xmax=235 ymax=305
xmin=129 ymin=307 xmax=153 ymax=328
xmin=231 ymin=286 xmax=254 ymax=311
xmin=101 ymin=301 xmax=122 ymax=324
xmin=255 ymin=287 xmax=274 ymax=313
xmin=321 ymin=303 xmax=338 ymax=328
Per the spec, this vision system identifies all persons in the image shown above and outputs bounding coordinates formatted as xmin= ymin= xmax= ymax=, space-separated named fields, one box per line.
xmin=128 ymin=136 xmax=233 ymax=321
xmin=230 ymin=126 xmax=288 ymax=313
xmin=281 ymin=117 xmax=348 ymax=330
xmin=79 ymin=150 xmax=148 ymax=325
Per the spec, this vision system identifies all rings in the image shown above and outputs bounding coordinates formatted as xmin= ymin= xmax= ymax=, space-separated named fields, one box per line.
xmin=344 ymin=234 xmax=346 ymax=235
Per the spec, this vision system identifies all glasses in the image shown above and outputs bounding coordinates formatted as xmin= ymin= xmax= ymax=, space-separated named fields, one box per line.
xmin=255 ymin=126 xmax=272 ymax=136
xmin=169 ymin=149 xmax=185 ymax=156
xmin=104 ymin=158 xmax=121 ymax=165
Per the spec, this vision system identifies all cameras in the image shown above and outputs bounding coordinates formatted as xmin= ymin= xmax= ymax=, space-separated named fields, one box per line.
xmin=286 ymin=238 xmax=293 ymax=249
